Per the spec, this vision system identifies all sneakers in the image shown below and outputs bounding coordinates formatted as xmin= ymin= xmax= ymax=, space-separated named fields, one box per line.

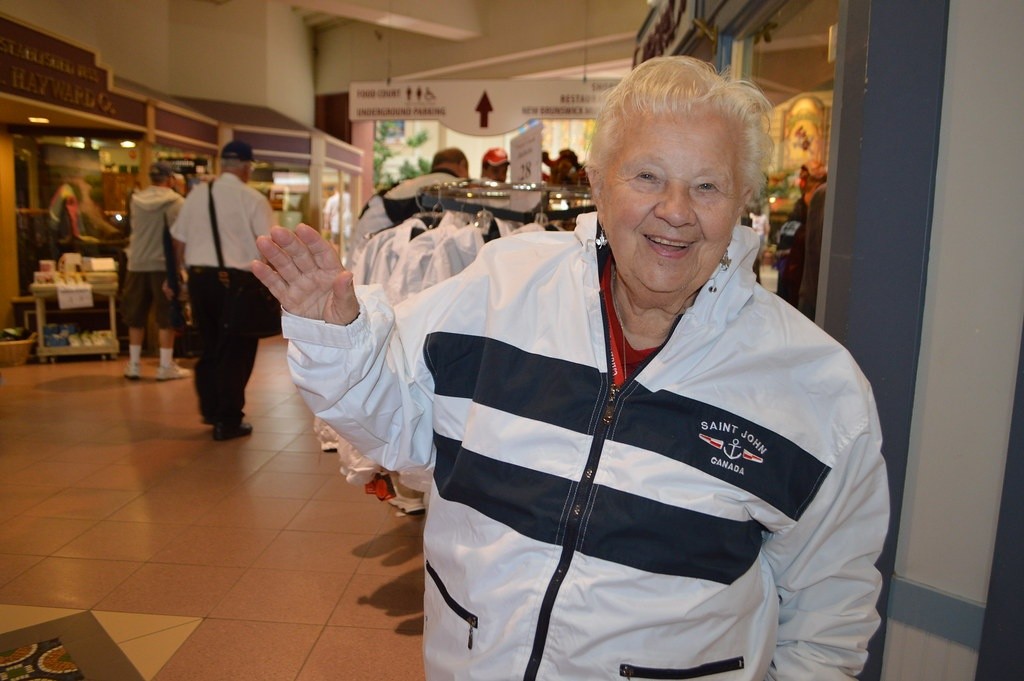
xmin=124 ymin=361 xmax=143 ymax=378
xmin=156 ymin=361 xmax=190 ymax=380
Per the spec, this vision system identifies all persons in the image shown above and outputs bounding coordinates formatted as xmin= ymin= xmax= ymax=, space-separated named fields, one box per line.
xmin=480 ymin=147 xmax=510 ymax=183
xmin=254 ymin=55 xmax=890 ymax=681
xmin=385 ymin=148 xmax=501 ymax=512
xmin=169 ymin=140 xmax=276 ymax=440
xmin=123 ymin=159 xmax=190 ymax=379
xmin=323 ymin=181 xmax=351 ymax=253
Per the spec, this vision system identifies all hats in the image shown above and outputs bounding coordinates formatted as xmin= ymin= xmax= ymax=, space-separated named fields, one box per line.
xmin=222 ymin=139 xmax=257 ymax=163
xmin=483 ymin=149 xmax=510 ymax=168
xmin=149 ymin=161 xmax=177 ymax=180
xmin=429 ymin=148 xmax=468 ymax=178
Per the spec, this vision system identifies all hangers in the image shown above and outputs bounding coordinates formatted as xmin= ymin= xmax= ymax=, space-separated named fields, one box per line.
xmin=475 ymin=195 xmax=494 ymax=229
xmin=428 ymin=190 xmax=445 ymax=228
xmin=413 ymin=188 xmax=431 ymax=218
xmin=456 ymin=195 xmax=472 ymax=225
xmin=535 ymin=206 xmax=553 ymax=227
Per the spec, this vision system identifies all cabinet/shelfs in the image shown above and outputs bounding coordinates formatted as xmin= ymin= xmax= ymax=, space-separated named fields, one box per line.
xmin=30 ymin=283 xmax=120 ymax=363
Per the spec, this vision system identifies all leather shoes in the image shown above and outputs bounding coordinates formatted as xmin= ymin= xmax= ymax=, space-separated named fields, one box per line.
xmin=214 ymin=422 xmax=251 ymax=440
xmin=202 ymin=411 xmax=244 ymax=425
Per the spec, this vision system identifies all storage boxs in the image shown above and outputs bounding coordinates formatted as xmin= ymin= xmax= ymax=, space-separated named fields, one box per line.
xmin=39 ymin=259 xmax=57 ymax=272
xmin=44 ymin=323 xmax=112 ymax=347
xmin=84 ymin=257 xmax=118 ymax=273
xmin=33 ymin=271 xmax=61 ymax=284
xmin=81 ymin=271 xmax=119 ymax=284
xmin=58 ymin=253 xmax=83 ymax=273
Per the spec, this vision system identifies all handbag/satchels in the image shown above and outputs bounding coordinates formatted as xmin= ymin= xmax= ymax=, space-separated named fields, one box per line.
xmin=222 ymin=274 xmax=282 ymax=339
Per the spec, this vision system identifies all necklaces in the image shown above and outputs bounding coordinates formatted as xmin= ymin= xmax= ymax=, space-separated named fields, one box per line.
xmin=612 ymin=271 xmax=626 ymax=380
